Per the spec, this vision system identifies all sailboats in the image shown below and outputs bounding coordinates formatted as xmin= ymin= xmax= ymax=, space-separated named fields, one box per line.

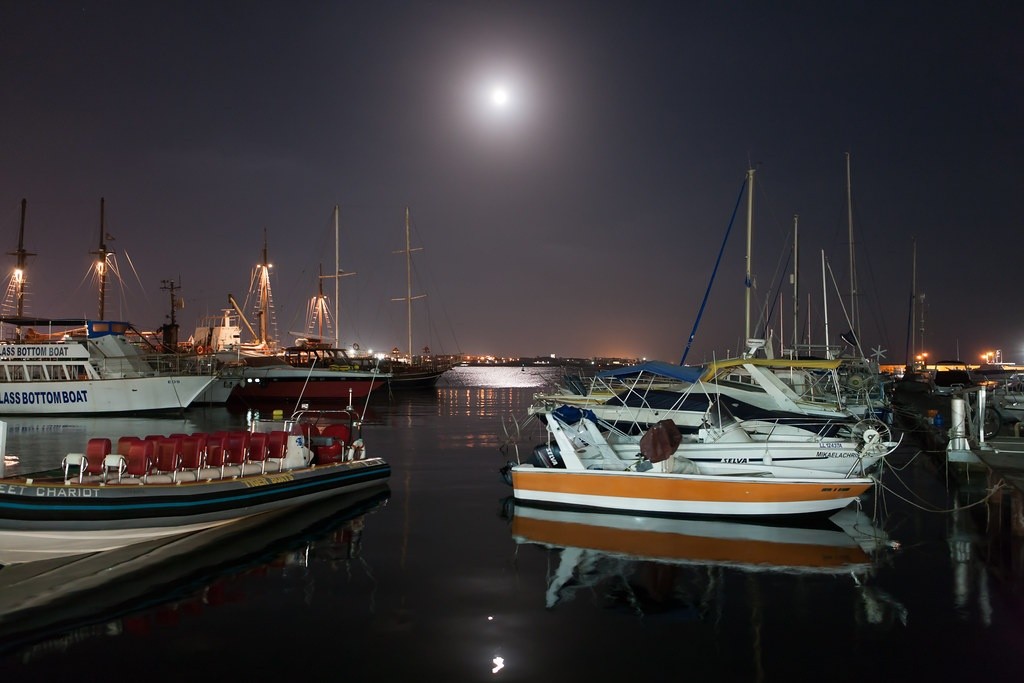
xmin=0 ymin=196 xmax=229 ymax=419
xmin=82 ymin=198 xmax=465 ymax=402
xmin=528 ymin=143 xmax=1023 ymax=479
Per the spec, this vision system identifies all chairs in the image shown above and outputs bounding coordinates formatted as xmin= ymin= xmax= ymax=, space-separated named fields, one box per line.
xmin=61 ymin=423 xmax=349 ymax=484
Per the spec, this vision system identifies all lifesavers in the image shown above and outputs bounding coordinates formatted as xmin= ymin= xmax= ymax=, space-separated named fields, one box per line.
xmin=197 ymin=345 xmax=204 ymax=355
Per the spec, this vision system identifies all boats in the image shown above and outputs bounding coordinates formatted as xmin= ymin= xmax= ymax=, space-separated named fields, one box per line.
xmin=0 ymin=353 xmax=398 ymax=635
xmin=505 ymin=494 xmax=910 ymax=628
xmin=497 ymin=350 xmax=878 ymax=523
